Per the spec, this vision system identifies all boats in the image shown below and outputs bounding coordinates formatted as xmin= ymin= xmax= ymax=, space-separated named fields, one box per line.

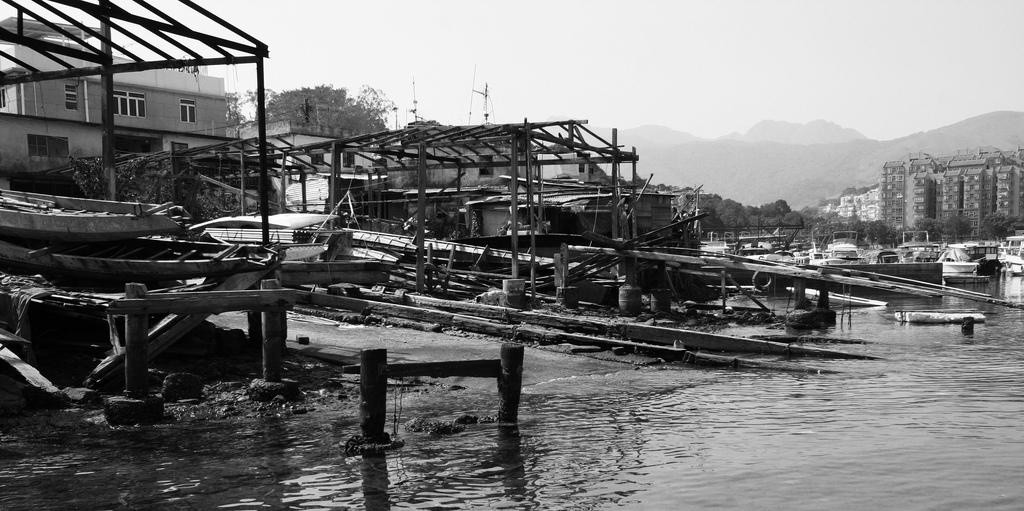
xmin=894 ymin=306 xmax=998 ymax=325
xmin=189 ymin=212 xmax=404 ymax=285
xmin=0 ymin=187 xmax=278 ymax=276
xmin=785 ymin=285 xmax=888 ymax=309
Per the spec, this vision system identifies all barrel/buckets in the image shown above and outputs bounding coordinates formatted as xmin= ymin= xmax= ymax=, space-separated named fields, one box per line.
xmin=556 ymin=285 xmax=579 ymax=308
xmin=651 ymin=287 xmax=670 ymax=314
xmin=620 ymin=284 xmax=641 ymax=313
xmin=503 ymin=278 xmax=525 ymax=308
